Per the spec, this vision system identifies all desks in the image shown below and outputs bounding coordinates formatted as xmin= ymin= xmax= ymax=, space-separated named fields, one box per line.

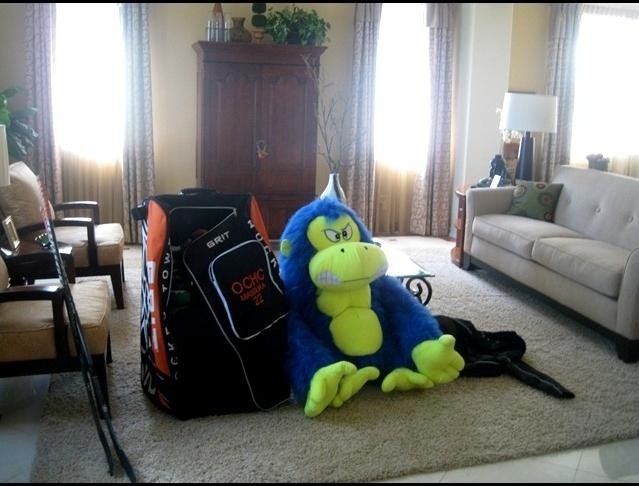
xmin=451 ymin=182 xmax=496 ymax=267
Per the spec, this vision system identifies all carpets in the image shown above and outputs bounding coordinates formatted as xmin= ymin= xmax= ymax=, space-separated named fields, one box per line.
xmin=29 ymin=234 xmax=639 ymax=484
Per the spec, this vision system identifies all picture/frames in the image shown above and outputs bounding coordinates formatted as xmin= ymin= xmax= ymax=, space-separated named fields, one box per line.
xmin=2 ymin=215 xmax=21 ymax=250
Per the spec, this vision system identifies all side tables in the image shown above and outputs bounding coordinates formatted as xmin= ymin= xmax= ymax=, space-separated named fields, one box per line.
xmin=0 ymin=237 xmax=72 ymax=279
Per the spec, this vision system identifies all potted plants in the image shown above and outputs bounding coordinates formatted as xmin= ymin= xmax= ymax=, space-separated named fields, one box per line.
xmin=265 ymin=4 xmax=332 ymax=45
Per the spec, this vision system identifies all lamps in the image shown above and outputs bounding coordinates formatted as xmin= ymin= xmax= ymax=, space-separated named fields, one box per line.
xmin=499 ymin=91 xmax=558 ymax=179
xmin=0 ymin=123 xmax=10 ymax=187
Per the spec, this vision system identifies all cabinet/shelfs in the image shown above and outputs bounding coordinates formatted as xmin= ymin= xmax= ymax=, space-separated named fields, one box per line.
xmin=190 ymin=41 xmax=328 ymax=250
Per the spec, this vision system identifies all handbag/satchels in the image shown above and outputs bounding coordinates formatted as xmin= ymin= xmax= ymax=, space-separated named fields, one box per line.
xmin=140 ymin=187 xmax=294 ymax=421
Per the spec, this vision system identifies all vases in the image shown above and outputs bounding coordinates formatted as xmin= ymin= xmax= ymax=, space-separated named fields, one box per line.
xmin=321 ymin=173 xmax=345 ymax=204
xmin=227 ymin=16 xmax=251 ymax=42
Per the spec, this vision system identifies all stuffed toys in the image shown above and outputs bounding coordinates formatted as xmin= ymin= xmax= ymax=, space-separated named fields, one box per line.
xmin=278 ymin=195 xmax=465 ymax=420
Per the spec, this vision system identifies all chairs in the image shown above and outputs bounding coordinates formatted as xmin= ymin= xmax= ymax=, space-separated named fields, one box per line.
xmin=0 ymin=257 xmax=114 ymax=421
xmin=0 ymin=162 xmax=125 ymax=310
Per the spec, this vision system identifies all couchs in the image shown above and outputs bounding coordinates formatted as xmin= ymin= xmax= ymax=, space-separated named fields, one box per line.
xmin=461 ymin=164 xmax=639 ymax=363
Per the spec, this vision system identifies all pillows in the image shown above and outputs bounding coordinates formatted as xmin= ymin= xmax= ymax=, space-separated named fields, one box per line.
xmin=507 ymin=178 xmax=564 ymax=222
